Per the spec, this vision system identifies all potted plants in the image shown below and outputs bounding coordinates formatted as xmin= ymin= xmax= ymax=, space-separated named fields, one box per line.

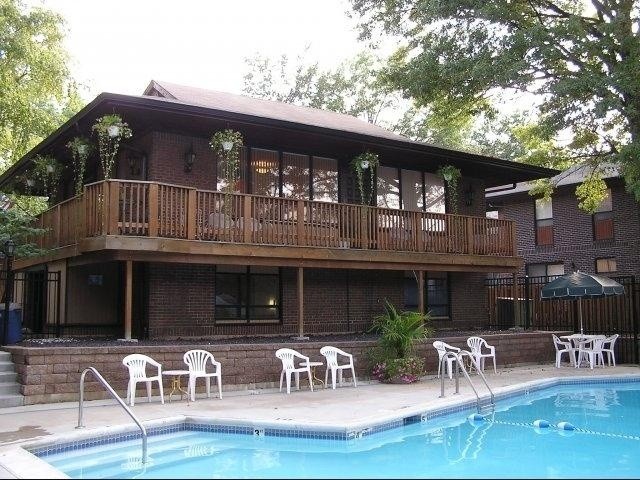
xmin=349 ymin=152 xmax=380 ymax=216
xmin=363 ymin=296 xmax=437 ymax=384
xmin=436 ymin=160 xmax=462 ymax=224
xmin=14 ymin=114 xmax=133 ymax=201
xmin=209 ymin=127 xmax=244 ymax=225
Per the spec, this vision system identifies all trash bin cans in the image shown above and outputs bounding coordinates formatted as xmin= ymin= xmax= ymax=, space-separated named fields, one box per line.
xmin=1 ymin=303 xmax=21 ymax=343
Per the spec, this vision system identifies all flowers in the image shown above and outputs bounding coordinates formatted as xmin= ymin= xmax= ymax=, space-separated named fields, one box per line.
xmin=370 ymin=356 xmax=427 ymax=384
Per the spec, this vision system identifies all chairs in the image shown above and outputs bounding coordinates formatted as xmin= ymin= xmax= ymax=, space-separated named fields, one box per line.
xmin=319 ymin=345 xmax=357 ymax=390
xmin=433 ymin=336 xmax=496 ymax=381
xmin=275 ymin=347 xmax=314 ymax=394
xmin=183 ymin=349 xmax=222 ymax=402
xmin=552 ymin=334 xmax=619 ymax=369
xmin=122 ymin=353 xmax=165 ymax=406
xmin=390 ymin=227 xmax=435 ymax=252
xmin=182 ymin=208 xmax=263 ymax=242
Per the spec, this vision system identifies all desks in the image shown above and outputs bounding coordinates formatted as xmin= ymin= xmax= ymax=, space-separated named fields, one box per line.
xmin=299 ymin=362 xmax=324 ymax=392
xmin=162 ymin=369 xmax=190 ymax=406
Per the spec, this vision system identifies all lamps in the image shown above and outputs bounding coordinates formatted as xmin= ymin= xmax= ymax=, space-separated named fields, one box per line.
xmin=184 ymin=142 xmax=197 ymax=172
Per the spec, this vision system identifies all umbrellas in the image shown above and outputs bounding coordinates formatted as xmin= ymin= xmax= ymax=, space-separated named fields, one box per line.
xmin=540 ymin=269 xmax=626 ymax=334
